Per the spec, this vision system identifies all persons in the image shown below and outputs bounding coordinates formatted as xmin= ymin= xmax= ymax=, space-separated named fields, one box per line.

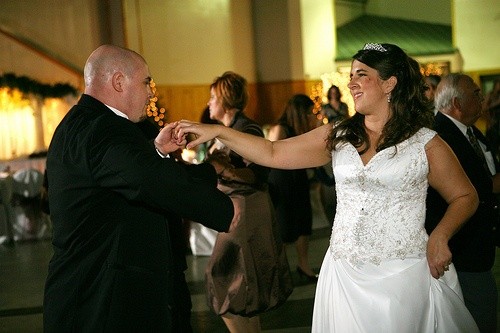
xmin=424 ymin=73 xmax=500 ymax=333
xmin=318 ymin=84 xmax=349 ymax=185
xmin=206 ymin=70 xmax=294 ymax=333
xmin=263 ymin=94 xmax=321 ymax=280
xmin=43 ymin=43 xmax=235 ymax=333
xmin=482 ymin=77 xmax=500 ymax=155
xmin=172 ymin=43 xmax=479 ymax=333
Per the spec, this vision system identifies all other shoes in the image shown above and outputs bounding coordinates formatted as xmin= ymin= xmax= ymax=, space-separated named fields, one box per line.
xmin=295 ymin=264 xmax=320 ymax=282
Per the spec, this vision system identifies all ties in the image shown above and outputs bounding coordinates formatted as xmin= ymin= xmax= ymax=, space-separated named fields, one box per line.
xmin=466 ymin=126 xmax=482 ymax=156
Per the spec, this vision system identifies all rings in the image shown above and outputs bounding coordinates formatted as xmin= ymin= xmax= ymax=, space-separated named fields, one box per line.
xmin=443 ymin=265 xmax=448 ymax=271
